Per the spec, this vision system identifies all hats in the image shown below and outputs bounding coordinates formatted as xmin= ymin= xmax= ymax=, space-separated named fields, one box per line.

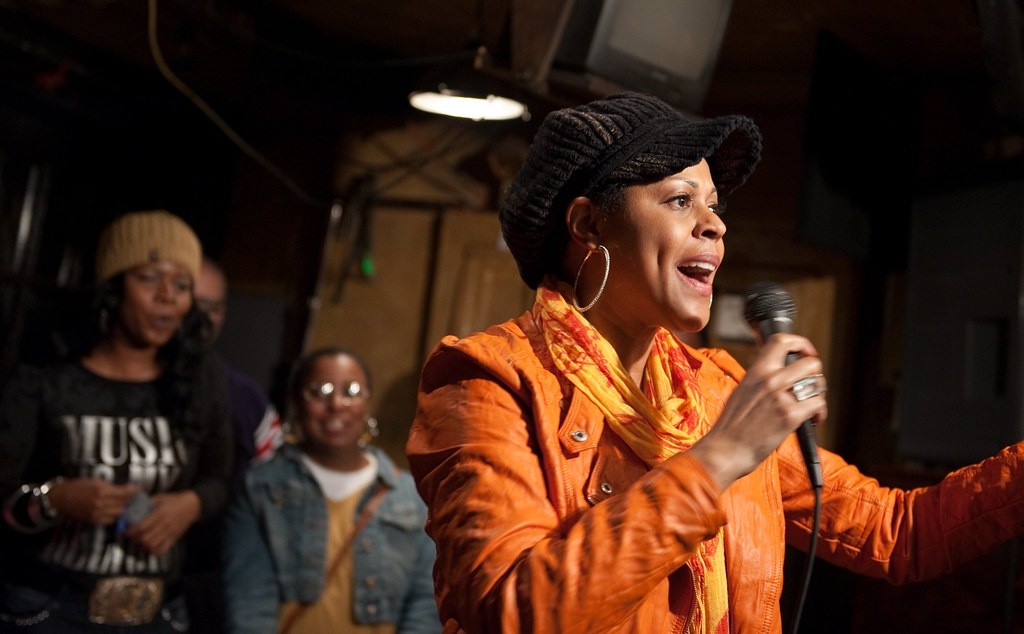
xmin=97 ymin=213 xmax=203 ymax=284
xmin=497 ymin=90 xmax=763 ymax=290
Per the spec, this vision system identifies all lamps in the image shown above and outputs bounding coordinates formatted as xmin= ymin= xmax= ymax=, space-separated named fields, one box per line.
xmin=407 ymin=0 xmax=529 ymax=123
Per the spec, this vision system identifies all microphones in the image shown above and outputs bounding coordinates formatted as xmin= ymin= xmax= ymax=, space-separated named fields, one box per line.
xmin=744 ymin=281 xmax=822 ymax=491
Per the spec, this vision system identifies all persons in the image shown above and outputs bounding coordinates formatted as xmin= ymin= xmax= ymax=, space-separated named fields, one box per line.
xmin=222 ymin=343 xmax=442 ymax=634
xmin=0 ymin=211 xmax=284 ymax=634
xmin=407 ymin=89 xmax=1024 ymax=634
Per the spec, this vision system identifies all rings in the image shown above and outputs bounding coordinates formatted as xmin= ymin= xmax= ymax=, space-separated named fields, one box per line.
xmin=792 ymin=375 xmax=820 ymax=402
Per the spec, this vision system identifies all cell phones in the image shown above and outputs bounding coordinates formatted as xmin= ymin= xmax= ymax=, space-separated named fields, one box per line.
xmin=122 ymin=493 xmax=152 ymax=524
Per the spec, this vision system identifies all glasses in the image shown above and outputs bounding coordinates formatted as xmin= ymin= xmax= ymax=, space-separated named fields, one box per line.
xmin=304 ymin=377 xmax=370 ymax=402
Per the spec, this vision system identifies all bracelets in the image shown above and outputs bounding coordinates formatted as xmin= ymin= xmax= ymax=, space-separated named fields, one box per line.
xmin=29 ymin=474 xmax=61 ymax=522
